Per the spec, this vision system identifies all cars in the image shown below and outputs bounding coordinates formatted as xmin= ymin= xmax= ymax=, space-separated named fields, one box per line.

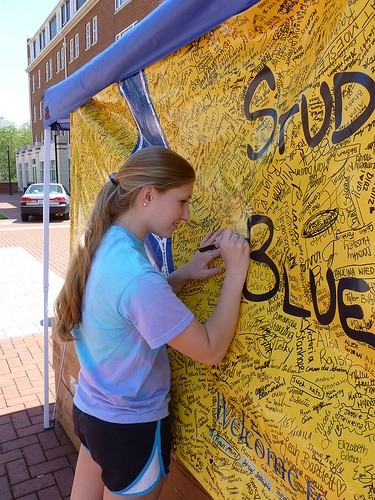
xmin=19 ymin=183 xmax=70 ymax=221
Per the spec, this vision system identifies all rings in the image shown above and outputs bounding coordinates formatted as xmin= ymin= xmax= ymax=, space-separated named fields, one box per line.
xmin=209 ymin=249 xmax=214 ymax=256
xmin=233 ymin=232 xmax=240 ymax=237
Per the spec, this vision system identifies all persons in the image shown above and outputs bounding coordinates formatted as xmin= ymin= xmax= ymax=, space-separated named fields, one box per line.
xmin=50 ymin=145 xmax=253 ymax=500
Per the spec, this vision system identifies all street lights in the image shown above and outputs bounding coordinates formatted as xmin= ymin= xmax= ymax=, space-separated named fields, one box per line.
xmin=7 ymin=144 xmax=13 ymax=196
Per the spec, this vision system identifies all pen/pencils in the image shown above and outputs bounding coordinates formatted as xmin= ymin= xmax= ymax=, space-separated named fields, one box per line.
xmin=199 ymin=243 xmax=220 ymax=253
